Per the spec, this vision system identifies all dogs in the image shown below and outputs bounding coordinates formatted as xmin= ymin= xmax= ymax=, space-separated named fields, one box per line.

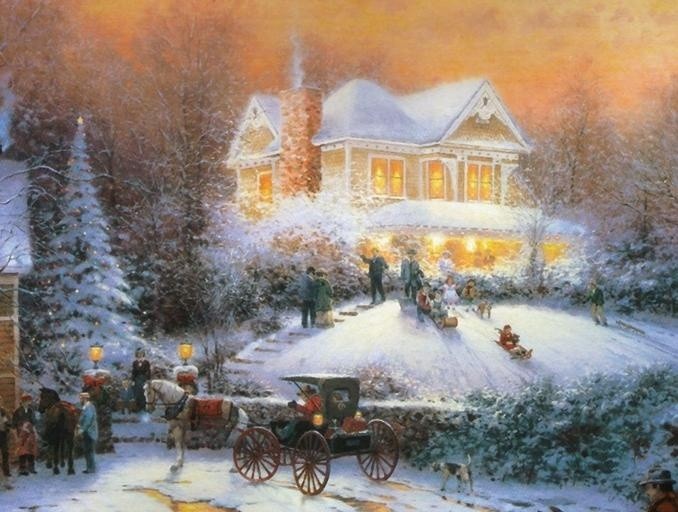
xmin=431 ymin=453 xmax=473 ymax=495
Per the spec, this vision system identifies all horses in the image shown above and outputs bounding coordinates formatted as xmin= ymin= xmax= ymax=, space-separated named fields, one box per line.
xmin=38 ymin=386 xmax=75 ymax=475
xmin=143 ymin=379 xmax=249 ymax=473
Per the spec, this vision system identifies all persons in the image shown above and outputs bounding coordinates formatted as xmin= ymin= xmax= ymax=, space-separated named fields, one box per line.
xmin=0 ymin=347 xmax=343 ymax=492
xmin=299 ymin=245 xmax=611 ymax=358
